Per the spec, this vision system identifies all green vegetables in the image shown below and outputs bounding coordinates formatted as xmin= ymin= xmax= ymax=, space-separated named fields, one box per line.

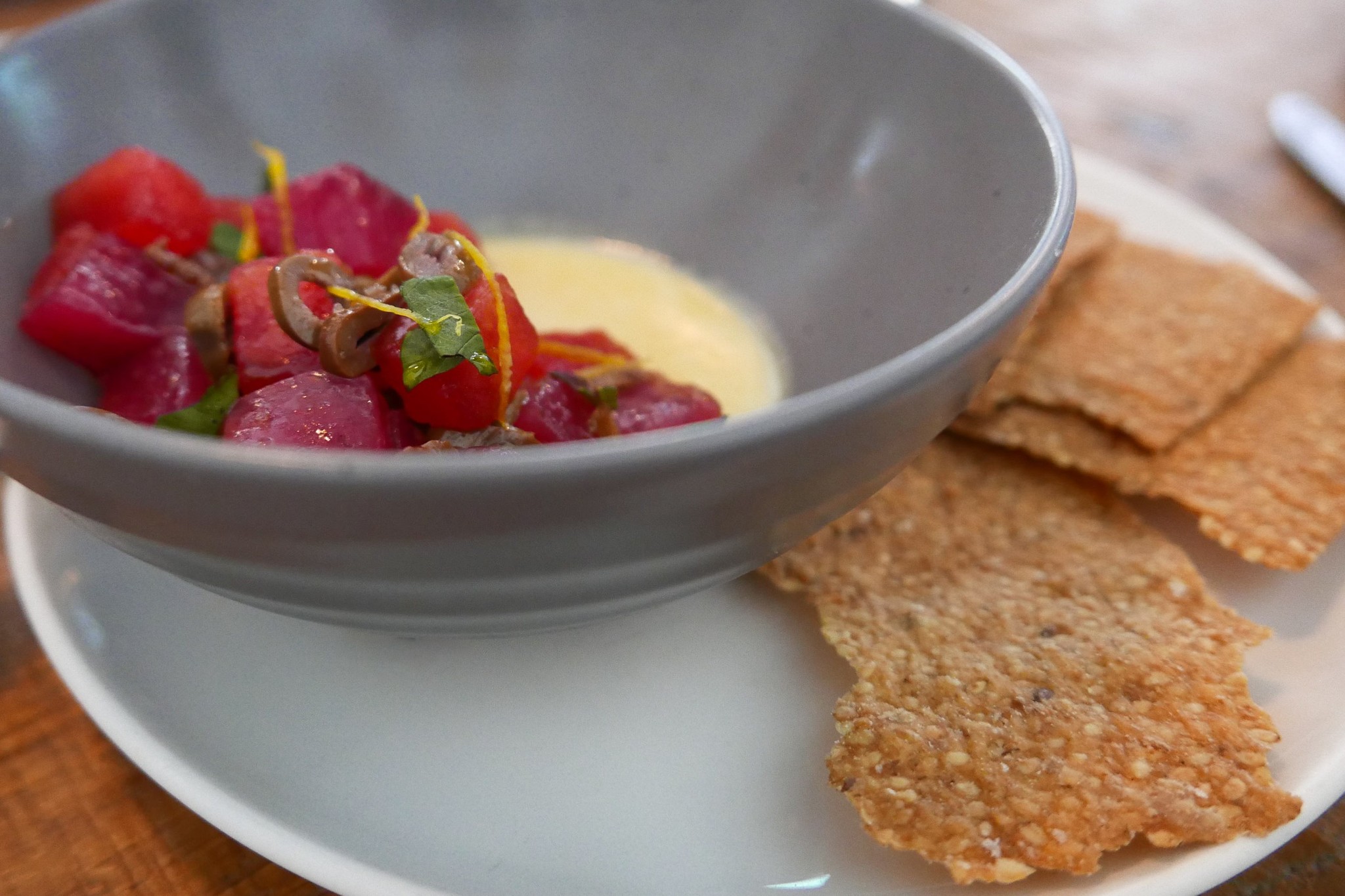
xmin=159 ymin=221 xmax=619 ymax=442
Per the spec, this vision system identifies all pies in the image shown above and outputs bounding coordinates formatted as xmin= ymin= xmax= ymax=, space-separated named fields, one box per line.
xmin=755 ymin=208 xmax=1344 ymax=883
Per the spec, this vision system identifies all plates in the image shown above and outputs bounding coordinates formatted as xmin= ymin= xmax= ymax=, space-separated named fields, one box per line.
xmin=0 ymin=148 xmax=1345 ymax=896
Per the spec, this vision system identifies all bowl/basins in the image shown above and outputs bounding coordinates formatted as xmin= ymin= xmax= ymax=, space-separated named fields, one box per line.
xmin=0 ymin=3 xmax=1079 ymax=646
